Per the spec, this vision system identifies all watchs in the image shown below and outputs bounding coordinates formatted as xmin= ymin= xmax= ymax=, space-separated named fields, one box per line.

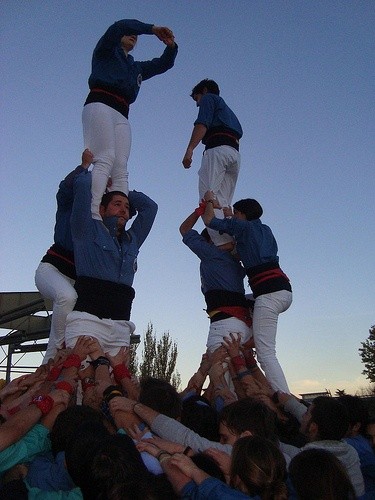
xmin=208 ymin=199 xmax=215 ymax=206
xmin=81 ymin=377 xmax=95 ymax=384
xmin=205 ymin=191 xmax=292 ymax=394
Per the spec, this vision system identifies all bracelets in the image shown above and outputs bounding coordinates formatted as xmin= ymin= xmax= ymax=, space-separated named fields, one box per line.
xmin=29 ymin=394 xmax=54 ymax=416
xmin=66 ymin=354 xmax=80 ymax=370
xmin=56 ymin=381 xmax=74 ymax=394
xmin=183 ymin=446 xmax=192 ymax=455
xmin=86 ymin=356 xmax=110 ymax=370
xmin=230 ymin=355 xmax=246 ymax=370
xmin=7 ymin=406 xmax=20 ymax=415
xmin=113 ymin=365 xmax=131 ymax=381
xmin=47 ymin=367 xmax=63 ymax=380
xmin=238 ymin=370 xmax=251 ymax=380
xmin=102 ymin=385 xmax=128 ymax=404
xmin=132 ymin=402 xmax=141 ymax=413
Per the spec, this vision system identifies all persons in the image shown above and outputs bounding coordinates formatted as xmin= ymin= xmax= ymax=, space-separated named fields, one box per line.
xmin=0 ymin=334 xmax=375 ymax=500
xmin=35 ymin=149 xmax=112 ymax=364
xmin=65 ymin=172 xmax=158 ymax=405
xmin=179 ymin=200 xmax=252 ymax=400
xmin=83 ymin=19 xmax=178 ymax=221
xmin=183 ymin=79 xmax=243 ymax=257
